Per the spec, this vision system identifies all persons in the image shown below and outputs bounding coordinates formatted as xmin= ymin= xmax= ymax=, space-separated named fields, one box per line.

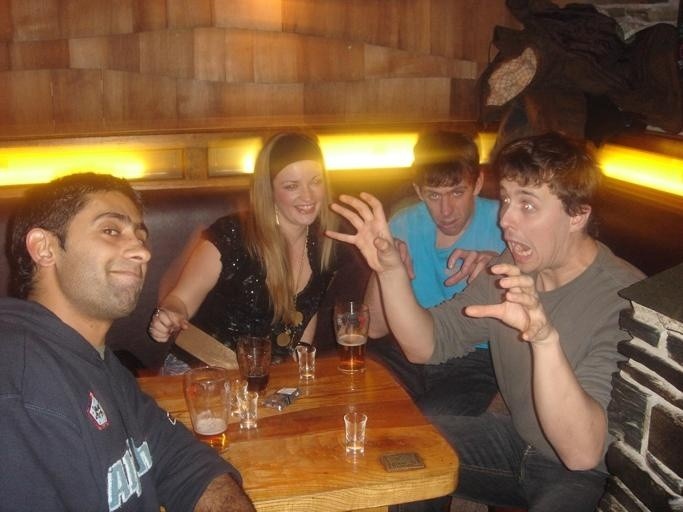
xmin=351 ymin=131 xmax=504 ymax=409
xmin=325 ymin=132 xmax=649 ymax=511
xmin=0 ymin=173 xmax=260 ymax=512
xmin=143 ymin=130 xmax=342 ymax=378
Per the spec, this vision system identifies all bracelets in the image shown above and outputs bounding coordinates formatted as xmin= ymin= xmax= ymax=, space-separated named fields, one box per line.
xmin=296 ymin=340 xmax=310 ymax=347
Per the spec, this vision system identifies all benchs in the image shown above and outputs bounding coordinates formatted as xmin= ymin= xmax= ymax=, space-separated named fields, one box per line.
xmin=0 ymin=164 xmax=683 ymax=376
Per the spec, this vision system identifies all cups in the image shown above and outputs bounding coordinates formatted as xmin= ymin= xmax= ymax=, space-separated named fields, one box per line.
xmin=181 ymin=300 xmax=369 ymax=458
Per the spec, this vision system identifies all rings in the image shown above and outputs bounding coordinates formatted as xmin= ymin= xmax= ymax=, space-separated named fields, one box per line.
xmin=155 ymin=309 xmax=163 ymax=317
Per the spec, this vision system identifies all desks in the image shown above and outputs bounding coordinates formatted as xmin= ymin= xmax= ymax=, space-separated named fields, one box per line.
xmin=132 ymin=357 xmax=460 ymax=512
